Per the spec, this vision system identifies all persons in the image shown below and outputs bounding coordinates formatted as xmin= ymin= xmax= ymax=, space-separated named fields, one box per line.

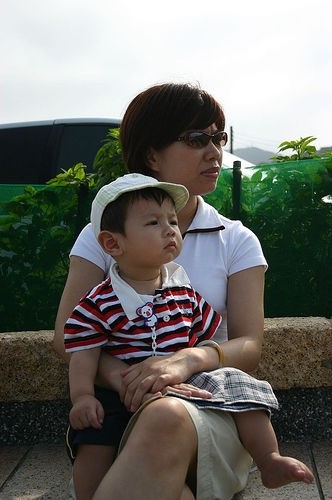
xmin=52 ymin=83 xmax=314 ymax=500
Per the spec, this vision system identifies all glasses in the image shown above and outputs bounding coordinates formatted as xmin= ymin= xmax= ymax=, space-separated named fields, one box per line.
xmin=175 ymin=131 xmax=228 ymax=150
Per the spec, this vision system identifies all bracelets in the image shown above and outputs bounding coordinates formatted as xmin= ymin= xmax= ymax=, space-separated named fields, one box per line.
xmin=196 ymin=340 xmax=224 ymax=370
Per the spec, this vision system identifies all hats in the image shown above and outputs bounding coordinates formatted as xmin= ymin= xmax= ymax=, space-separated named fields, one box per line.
xmin=90 ymin=173 xmax=190 ymax=251
